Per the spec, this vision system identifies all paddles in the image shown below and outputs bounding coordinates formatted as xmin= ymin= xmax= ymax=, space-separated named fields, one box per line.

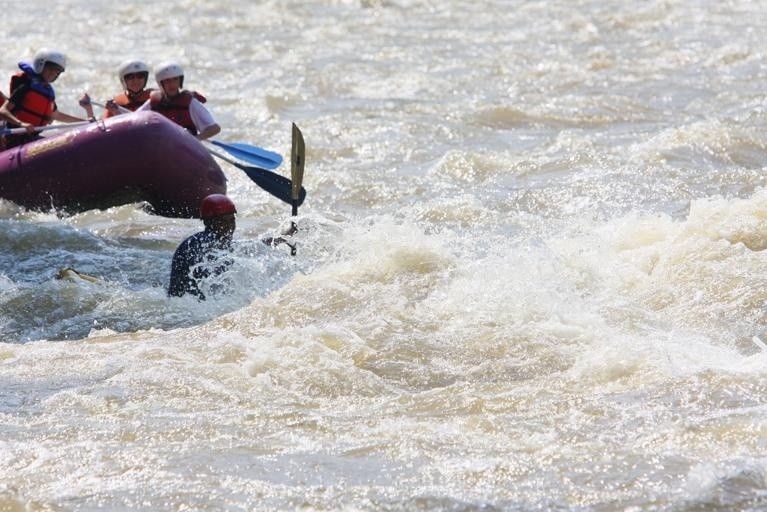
xmin=289 ymin=123 xmax=305 ymax=261
xmin=117 ymin=107 xmax=283 ymax=169
xmin=85 ymin=101 xmax=306 ymax=206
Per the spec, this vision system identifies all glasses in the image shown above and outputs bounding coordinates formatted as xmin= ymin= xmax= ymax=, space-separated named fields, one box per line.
xmin=126 ymin=73 xmax=144 ymax=79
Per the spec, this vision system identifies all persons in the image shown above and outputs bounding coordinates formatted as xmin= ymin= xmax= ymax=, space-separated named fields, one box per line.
xmin=105 ymin=60 xmax=221 ymax=141
xmin=0 ymin=48 xmax=95 ymax=153
xmin=79 ymin=58 xmax=161 ymax=122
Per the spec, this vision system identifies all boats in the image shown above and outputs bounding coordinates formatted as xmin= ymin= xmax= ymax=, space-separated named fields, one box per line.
xmin=0 ymin=109 xmax=228 ymax=220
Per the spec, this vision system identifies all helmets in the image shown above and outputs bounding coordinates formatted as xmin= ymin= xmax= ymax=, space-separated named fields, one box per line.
xmin=118 ymin=60 xmax=150 ymax=89
xmin=200 ymin=194 xmax=237 ymax=225
xmin=33 ymin=48 xmax=65 ymax=75
xmin=155 ymin=62 xmax=184 ymax=98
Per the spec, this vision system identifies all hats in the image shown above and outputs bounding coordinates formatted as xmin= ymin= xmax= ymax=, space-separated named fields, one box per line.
xmin=45 ymin=61 xmax=64 ymax=72
